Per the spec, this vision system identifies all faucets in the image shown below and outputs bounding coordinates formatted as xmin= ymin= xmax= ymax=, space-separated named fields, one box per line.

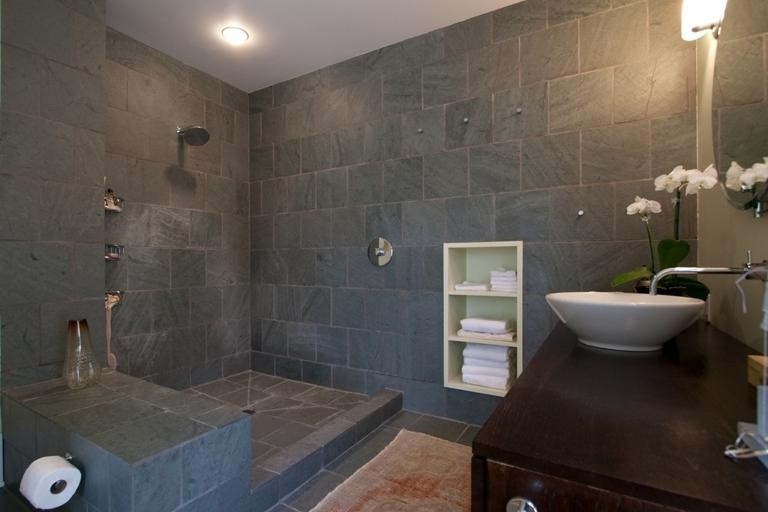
xmin=645 ymin=250 xmax=767 ymax=299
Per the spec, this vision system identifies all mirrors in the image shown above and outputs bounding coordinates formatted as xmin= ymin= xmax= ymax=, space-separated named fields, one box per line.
xmin=707 ymin=0 xmax=768 ymax=210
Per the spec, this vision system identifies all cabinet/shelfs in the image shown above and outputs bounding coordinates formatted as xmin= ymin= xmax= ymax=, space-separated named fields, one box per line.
xmin=441 ymin=240 xmax=524 ymax=397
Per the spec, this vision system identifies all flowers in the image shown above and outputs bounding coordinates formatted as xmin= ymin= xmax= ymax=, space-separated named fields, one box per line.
xmin=624 ymin=164 xmax=718 ymax=284
xmin=723 ymin=154 xmax=768 ymax=195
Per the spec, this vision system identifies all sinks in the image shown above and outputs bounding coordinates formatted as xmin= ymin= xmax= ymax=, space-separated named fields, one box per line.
xmin=545 ymin=287 xmax=705 ymax=353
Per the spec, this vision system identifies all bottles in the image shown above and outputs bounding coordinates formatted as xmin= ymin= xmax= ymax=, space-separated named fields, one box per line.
xmin=63 ymin=318 xmax=99 ymax=388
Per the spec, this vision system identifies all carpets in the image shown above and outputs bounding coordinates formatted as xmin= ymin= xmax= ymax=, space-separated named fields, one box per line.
xmin=307 ymin=425 xmax=471 ymax=511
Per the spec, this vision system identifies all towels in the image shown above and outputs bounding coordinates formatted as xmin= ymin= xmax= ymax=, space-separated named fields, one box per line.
xmin=454 ymin=268 xmax=517 ymax=392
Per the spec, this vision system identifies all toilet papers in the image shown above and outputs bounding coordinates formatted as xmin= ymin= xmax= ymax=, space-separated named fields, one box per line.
xmin=19 ymin=455 xmax=83 ymax=512
